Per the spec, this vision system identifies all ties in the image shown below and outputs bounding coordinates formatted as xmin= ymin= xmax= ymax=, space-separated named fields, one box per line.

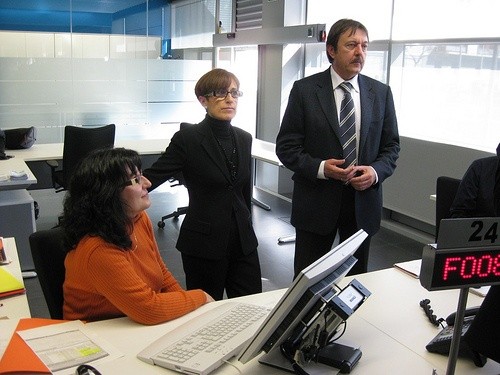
xmin=337 ymin=83 xmax=357 ymax=184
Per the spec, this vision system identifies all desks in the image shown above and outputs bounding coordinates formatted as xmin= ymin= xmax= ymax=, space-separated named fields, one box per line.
xmin=0 ymin=138 xmax=500 ymax=375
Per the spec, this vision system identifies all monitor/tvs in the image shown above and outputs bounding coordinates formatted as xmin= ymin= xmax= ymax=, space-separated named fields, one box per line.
xmin=238 ymin=228 xmax=370 ymax=375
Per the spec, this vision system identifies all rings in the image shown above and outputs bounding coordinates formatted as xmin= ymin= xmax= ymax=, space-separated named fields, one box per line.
xmin=341 ymin=177 xmax=342 ymax=180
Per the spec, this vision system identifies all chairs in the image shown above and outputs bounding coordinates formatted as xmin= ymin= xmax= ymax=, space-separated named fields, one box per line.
xmin=157 ymin=122 xmax=195 ymax=229
xmin=46 ymin=124 xmax=116 ymax=194
xmin=29 ymin=228 xmax=72 ymax=320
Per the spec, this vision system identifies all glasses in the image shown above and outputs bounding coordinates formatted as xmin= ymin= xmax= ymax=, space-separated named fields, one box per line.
xmin=120 ymin=169 xmax=143 ymax=188
xmin=204 ymin=88 xmax=243 ymax=97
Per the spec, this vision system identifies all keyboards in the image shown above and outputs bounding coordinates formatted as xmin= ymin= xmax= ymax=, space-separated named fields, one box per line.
xmin=136 ymin=301 xmax=271 ymax=375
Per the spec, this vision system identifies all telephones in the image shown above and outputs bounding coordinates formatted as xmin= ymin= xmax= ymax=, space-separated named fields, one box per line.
xmin=426 ymin=306 xmax=488 ymax=367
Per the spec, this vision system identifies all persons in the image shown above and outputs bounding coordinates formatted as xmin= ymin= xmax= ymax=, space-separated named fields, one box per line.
xmin=142 ymin=68 xmax=262 ymax=301
xmin=276 ymin=19 xmax=402 ymax=283
xmin=58 ymin=147 xmax=215 ymax=326
xmin=447 ymin=143 xmax=500 ymax=219
